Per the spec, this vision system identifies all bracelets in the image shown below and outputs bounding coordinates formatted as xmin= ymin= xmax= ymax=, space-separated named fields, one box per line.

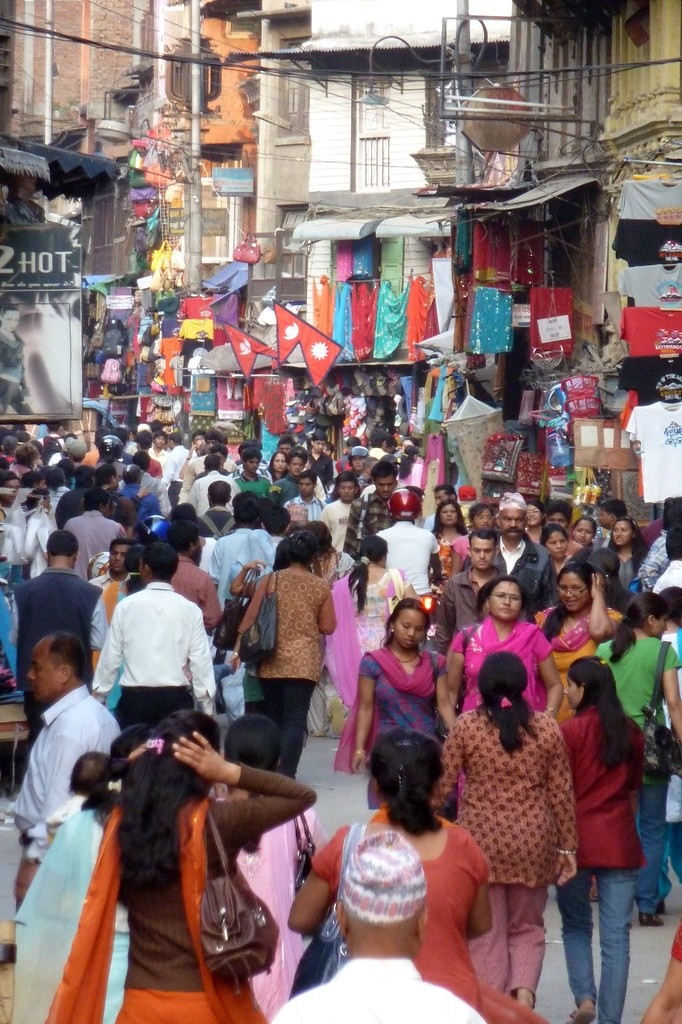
xmin=557 ymin=849 xmax=577 ymax=855
xmin=545 ymin=708 xmax=558 ymax=715
xmin=353 ymin=749 xmax=367 ymax=755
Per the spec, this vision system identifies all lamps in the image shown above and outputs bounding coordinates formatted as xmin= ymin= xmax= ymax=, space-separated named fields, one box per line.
xmin=251 ymin=109 xmax=311 ymax=146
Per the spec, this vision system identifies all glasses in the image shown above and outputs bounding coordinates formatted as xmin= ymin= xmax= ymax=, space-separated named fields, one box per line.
xmin=526 ymin=508 xmax=541 ymax=515
xmin=555 ymin=583 xmax=591 ymax=597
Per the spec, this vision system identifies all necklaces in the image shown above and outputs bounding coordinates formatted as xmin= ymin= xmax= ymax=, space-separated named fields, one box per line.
xmin=399 ymin=657 xmax=418 ymax=663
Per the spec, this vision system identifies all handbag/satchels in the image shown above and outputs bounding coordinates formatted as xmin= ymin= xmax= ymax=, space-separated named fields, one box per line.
xmin=288 ymin=823 xmax=366 ymax=999
xmin=79 ymin=136 xmax=305 ymax=425
xmin=213 ymin=566 xmax=260 ymax=651
xmin=199 ymin=810 xmax=278 ymax=997
xmin=642 ymin=640 xmax=682 ymax=782
xmin=293 ymin=811 xmax=342 ymax=943
xmin=430 ymin=650 xmax=451 ymax=744
xmin=239 ymin=571 xmax=278 ymax=668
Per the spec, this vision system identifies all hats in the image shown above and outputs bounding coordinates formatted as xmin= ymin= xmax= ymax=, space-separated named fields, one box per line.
xmin=281 ymin=364 xmax=410 ymax=446
xmin=458 ymin=486 xmax=477 ymax=501
xmin=342 ymin=831 xmax=427 ymax=925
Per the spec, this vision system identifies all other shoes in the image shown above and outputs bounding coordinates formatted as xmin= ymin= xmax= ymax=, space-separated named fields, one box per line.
xmin=638 ymin=912 xmax=664 ymax=926
xmin=313 ymin=732 xmax=326 ymax=738
xmin=656 ymin=903 xmax=666 ymax=913
xmin=566 ymin=1007 xmax=596 ymax=1024
xmin=328 ymin=698 xmax=345 ymax=737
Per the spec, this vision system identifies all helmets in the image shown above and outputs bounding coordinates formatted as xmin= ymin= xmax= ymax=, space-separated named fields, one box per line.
xmin=387 ymin=488 xmax=423 ymax=520
xmin=65 ymin=438 xmax=88 ymax=459
xmin=136 ymin=515 xmax=171 ymax=542
xmin=98 ymin=434 xmax=124 ymax=459
xmin=348 ymin=446 xmax=370 ymax=467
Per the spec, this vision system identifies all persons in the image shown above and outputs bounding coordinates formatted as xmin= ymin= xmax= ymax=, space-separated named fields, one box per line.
xmin=560 ymin=655 xmax=649 ymax=1024
xmin=431 ymin=651 xmax=578 ymax=1009
xmin=0 ymin=416 xmax=682 ymax=1024
